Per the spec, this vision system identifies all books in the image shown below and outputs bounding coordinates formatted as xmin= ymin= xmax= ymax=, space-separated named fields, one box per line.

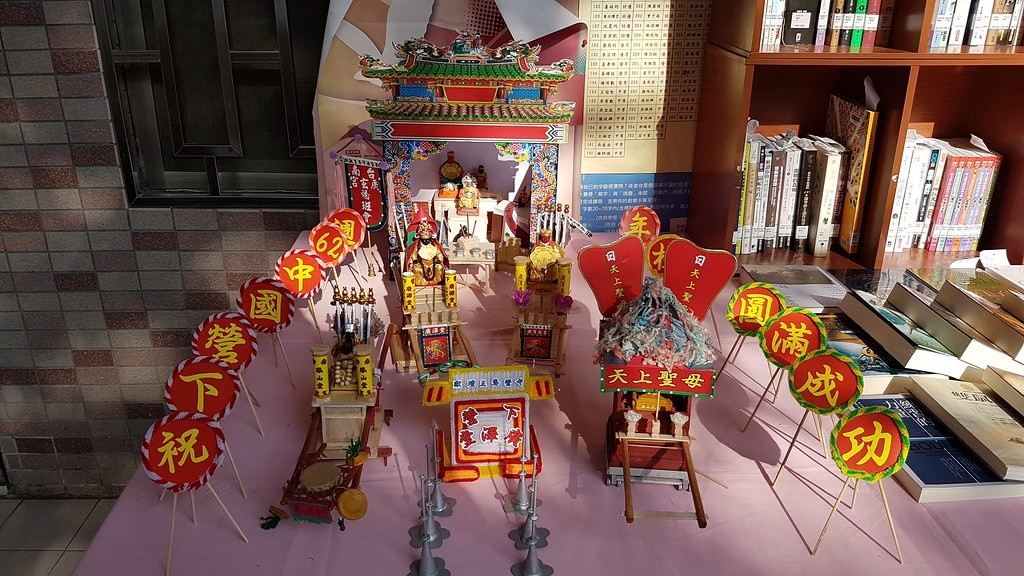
xmin=734 ymin=90 xmax=880 ymax=259
xmin=929 ymin=0 xmax=1024 ymax=53
xmin=880 ymin=121 xmax=1004 ymax=252
xmin=808 ymin=265 xmax=1024 ymax=504
xmin=758 ymin=0 xmax=896 ymax=55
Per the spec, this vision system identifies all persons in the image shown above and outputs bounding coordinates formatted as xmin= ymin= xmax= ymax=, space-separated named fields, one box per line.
xmin=529 ymin=229 xmax=565 ymax=282
xmin=439 ymin=150 xmax=529 ymax=210
xmin=406 ymin=217 xmax=451 ymax=286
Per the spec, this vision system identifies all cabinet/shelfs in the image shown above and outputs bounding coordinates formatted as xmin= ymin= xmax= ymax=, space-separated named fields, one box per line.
xmin=686 ymin=0 xmax=1024 ymax=269
